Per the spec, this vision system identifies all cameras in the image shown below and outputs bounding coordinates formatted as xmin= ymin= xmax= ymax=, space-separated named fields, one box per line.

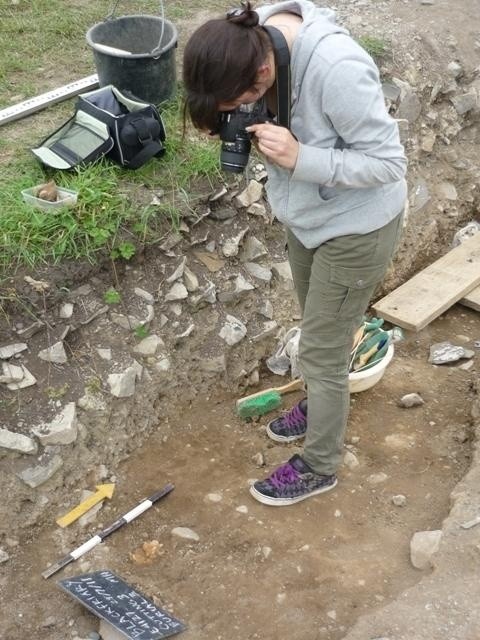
xmin=202 ymin=110 xmax=275 ymax=173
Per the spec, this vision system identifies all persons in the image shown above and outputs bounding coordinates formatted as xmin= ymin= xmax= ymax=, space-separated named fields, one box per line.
xmin=181 ymin=1 xmax=408 ymax=508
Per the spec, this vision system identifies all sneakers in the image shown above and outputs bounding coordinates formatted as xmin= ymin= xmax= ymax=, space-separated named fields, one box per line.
xmin=250 ymin=454 xmax=338 ymax=506
xmin=266 ymin=396 xmax=307 ymax=443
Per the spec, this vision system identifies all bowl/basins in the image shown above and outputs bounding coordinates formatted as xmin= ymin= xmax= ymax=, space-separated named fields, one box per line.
xmin=348 ymin=320 xmax=394 ymax=393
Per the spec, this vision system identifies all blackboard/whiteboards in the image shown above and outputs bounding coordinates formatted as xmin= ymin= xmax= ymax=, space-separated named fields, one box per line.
xmin=56 ymin=570 xmax=186 ymax=640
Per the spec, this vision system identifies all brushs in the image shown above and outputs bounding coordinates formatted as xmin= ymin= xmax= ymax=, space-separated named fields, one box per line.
xmin=235 ymin=378 xmax=305 ymax=419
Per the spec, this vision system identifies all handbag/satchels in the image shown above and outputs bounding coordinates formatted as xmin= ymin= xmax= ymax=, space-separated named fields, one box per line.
xmin=29 ymin=84 xmax=166 ymax=171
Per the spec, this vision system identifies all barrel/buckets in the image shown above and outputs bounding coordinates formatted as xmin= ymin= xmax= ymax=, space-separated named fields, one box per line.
xmin=85 ymin=1 xmax=178 ymax=107
